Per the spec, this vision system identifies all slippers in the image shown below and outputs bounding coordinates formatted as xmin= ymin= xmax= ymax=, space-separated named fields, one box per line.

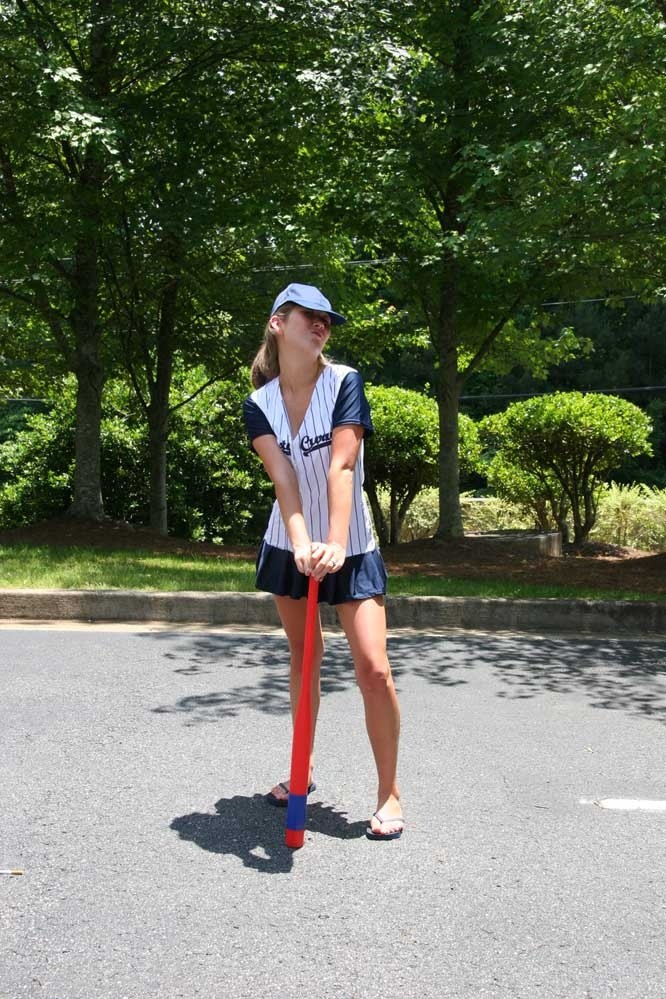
xmin=267 ymin=770 xmax=317 ymax=806
xmin=366 ymin=805 xmax=405 ymax=840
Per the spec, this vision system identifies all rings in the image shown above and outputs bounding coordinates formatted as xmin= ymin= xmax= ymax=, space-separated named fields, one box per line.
xmin=330 ymin=559 xmax=337 ymax=567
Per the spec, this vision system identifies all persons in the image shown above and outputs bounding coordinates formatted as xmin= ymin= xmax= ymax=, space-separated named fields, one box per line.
xmin=242 ymin=283 xmax=407 ymax=839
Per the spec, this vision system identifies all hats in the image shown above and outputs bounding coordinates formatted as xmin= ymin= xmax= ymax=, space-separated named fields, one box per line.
xmin=271 ymin=284 xmax=346 ymax=326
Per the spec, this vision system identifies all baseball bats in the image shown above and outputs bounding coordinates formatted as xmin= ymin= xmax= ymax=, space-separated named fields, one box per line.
xmin=285 ymin=575 xmax=318 ymax=848
